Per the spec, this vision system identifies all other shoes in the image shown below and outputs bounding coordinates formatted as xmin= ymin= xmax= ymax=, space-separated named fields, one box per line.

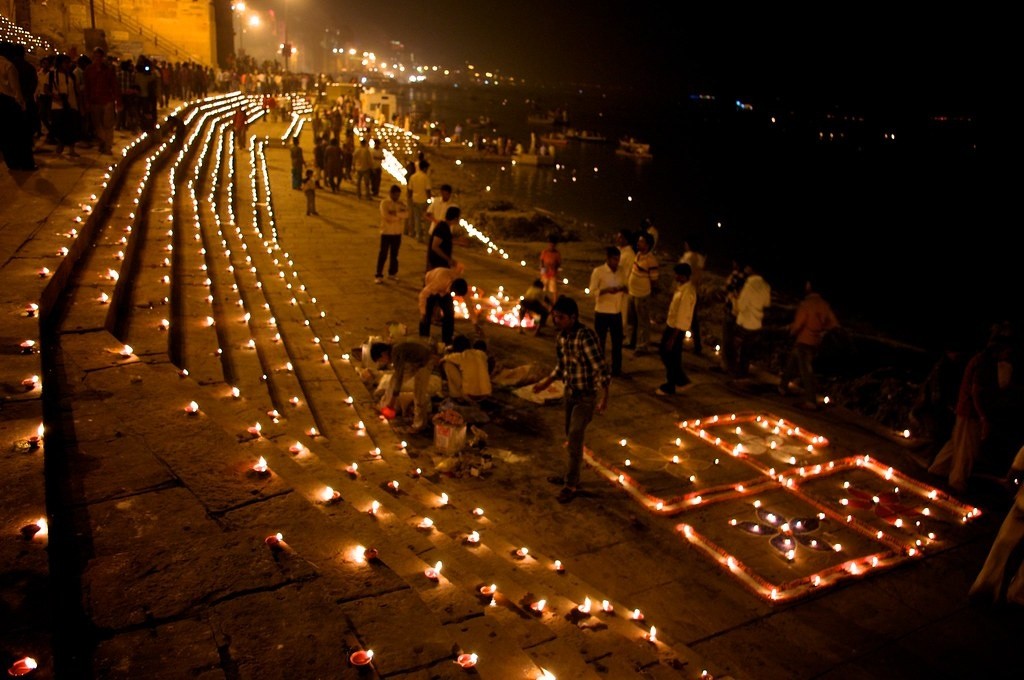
xmin=634 ymin=347 xmax=646 ymax=357
xmin=796 ymin=401 xmax=819 ymax=410
xmin=388 ymin=275 xmax=400 ymax=281
xmin=547 ymin=475 xmax=567 ymax=485
xmin=610 ymin=370 xmax=633 ymax=381
xmin=375 ymin=277 xmax=383 ymax=284
xmin=676 ymin=381 xmax=694 ymax=391
xmin=622 ymin=342 xmax=636 ymax=349
xmin=555 ymin=487 xmax=578 ymax=504
xmin=656 ymin=389 xmax=670 ymax=396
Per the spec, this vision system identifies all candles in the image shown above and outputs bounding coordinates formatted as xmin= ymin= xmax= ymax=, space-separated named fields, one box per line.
xmin=22 ymin=340 xmax=35 ymax=350
xmin=58 ymin=165 xmax=984 ymax=628
xmin=39 ymin=267 xmax=50 ymax=276
xmin=9 ymin=657 xmax=37 ymax=675
xmin=29 ymin=422 xmax=44 ymax=448
xmin=645 ymin=626 xmax=656 ymax=642
xmin=26 ymin=303 xmax=38 ymax=315
xmin=350 ymin=649 xmax=375 ymax=665
xmin=22 ymin=375 xmax=38 ymax=386
xmin=456 ymin=653 xmax=479 ymax=667
xmin=20 ymin=517 xmax=46 ymax=538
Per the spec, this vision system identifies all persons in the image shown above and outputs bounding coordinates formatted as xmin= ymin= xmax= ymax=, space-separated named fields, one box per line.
xmin=0 ymin=42 xmax=495 ymax=434
xmin=518 ymin=211 xmax=1024 ymax=609
